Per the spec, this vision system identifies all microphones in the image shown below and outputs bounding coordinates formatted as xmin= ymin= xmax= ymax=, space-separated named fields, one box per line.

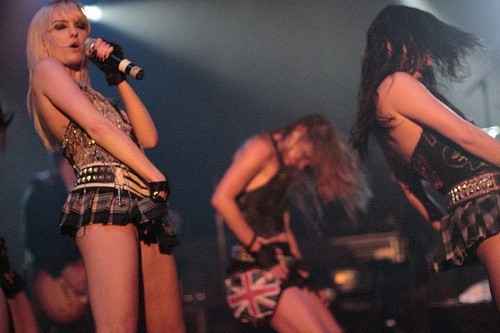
xmin=84 ymin=37 xmax=144 ymax=81
